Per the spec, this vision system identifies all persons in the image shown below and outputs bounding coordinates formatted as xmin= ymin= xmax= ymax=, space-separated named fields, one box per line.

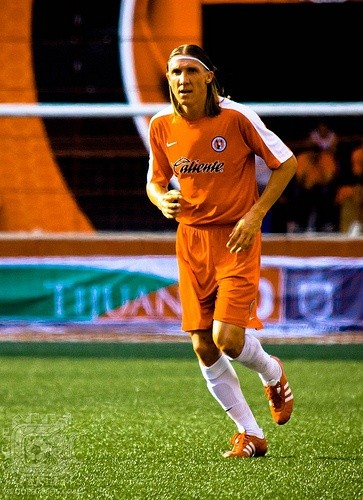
xmin=256 ymin=153 xmax=282 ymax=233
xmin=146 ymin=43 xmax=299 ymax=457
xmin=296 ymin=144 xmax=337 ymax=234
xmin=306 ymin=122 xmax=338 ymax=155
xmin=350 ymin=148 xmax=363 ymax=205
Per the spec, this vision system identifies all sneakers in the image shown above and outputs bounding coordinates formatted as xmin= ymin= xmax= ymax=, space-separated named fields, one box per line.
xmin=262 ymin=354 xmax=294 ymax=426
xmin=222 ymin=429 xmax=268 ymax=458
